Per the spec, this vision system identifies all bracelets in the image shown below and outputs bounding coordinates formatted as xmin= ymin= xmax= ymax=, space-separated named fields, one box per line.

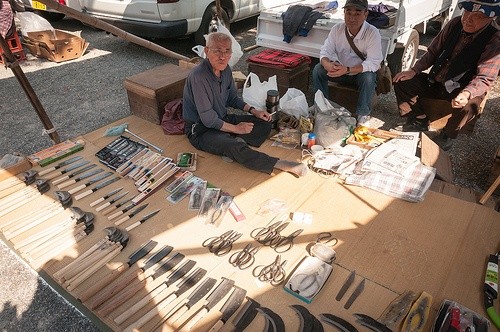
xmin=347 ymin=67 xmax=349 ymax=74
xmin=248 ymin=107 xmax=255 ymax=114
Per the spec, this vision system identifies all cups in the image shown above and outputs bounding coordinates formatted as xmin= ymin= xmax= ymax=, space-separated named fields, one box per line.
xmin=311 ymin=145 xmax=323 ymax=154
xmin=301 ymin=132 xmax=310 ymax=146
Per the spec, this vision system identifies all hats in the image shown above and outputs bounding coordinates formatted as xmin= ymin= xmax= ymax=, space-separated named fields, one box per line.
xmin=343 ymin=0 xmax=368 ymax=11
xmin=458 ymin=0 xmax=500 ymax=18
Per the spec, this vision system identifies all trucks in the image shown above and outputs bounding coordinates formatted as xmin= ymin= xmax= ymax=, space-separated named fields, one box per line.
xmin=254 ymin=0 xmax=471 ymax=86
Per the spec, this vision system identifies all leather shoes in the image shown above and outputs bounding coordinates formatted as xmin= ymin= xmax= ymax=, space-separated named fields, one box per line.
xmin=394 ymin=117 xmax=427 ymax=131
xmin=433 ymin=129 xmax=453 ymax=151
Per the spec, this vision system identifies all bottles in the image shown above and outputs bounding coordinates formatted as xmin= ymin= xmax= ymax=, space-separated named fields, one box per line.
xmin=307 ymin=133 xmax=316 ymax=149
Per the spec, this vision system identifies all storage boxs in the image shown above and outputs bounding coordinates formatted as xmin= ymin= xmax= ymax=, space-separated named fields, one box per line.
xmin=228 ymin=201 xmax=246 ymax=222
xmin=124 ymin=63 xmax=193 ymax=123
xmin=326 ymin=81 xmax=382 ymax=112
xmin=483 ymin=146 xmax=500 ymax=196
xmin=284 ymin=256 xmax=334 ymax=304
xmin=0 ymin=30 xmax=26 ymax=65
xmin=247 ymin=61 xmax=310 ymax=94
xmin=347 ymin=131 xmax=389 ymax=150
xmin=27 ymin=140 xmax=84 ymax=167
xmin=232 ymin=71 xmax=247 ymax=90
xmin=424 ymin=97 xmax=478 ymax=134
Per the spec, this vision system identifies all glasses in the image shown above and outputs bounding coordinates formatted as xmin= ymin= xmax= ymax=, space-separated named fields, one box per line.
xmin=207 ymin=47 xmax=232 ymax=57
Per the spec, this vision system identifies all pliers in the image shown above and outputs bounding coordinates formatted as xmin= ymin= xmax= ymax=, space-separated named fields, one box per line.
xmin=209 ymin=196 xmax=230 ymax=224
xmin=380 ymin=286 xmax=415 ymax=327
xmin=199 ymin=188 xmax=219 ymax=216
xmin=166 ymin=171 xmax=193 ymax=192
xmin=403 ymin=296 xmax=430 ymax=332
xmin=170 ymin=177 xmax=200 ymax=201
xmin=190 ymin=182 xmax=206 ymax=209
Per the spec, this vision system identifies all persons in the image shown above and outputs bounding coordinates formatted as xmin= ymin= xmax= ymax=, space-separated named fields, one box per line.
xmin=184 ymin=33 xmax=307 ymax=177
xmin=392 ymin=0 xmax=500 ymax=150
xmin=313 ymin=0 xmax=382 ymax=118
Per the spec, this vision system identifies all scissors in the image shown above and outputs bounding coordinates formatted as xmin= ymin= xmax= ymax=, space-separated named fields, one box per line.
xmin=288 ymin=263 xmax=326 ymax=298
xmin=309 ymin=230 xmax=339 ymax=248
xmin=251 ymin=254 xmax=288 ymax=288
xmin=258 ymin=222 xmax=291 ymax=246
xmin=249 ymin=219 xmax=283 ymax=243
xmin=227 ymin=241 xmax=262 ymax=270
xmin=269 ymin=228 xmax=304 ymax=253
xmin=201 ymin=229 xmax=244 ymax=257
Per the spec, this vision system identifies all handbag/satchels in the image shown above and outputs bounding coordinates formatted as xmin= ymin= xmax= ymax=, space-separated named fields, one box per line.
xmin=377 ymin=65 xmax=392 ymax=95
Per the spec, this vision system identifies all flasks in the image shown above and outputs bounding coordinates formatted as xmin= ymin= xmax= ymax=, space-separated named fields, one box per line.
xmin=266 ymin=90 xmax=280 ymax=114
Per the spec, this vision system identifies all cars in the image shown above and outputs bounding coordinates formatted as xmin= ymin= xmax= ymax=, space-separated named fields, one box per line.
xmin=7 ymin=0 xmax=334 ymax=50
